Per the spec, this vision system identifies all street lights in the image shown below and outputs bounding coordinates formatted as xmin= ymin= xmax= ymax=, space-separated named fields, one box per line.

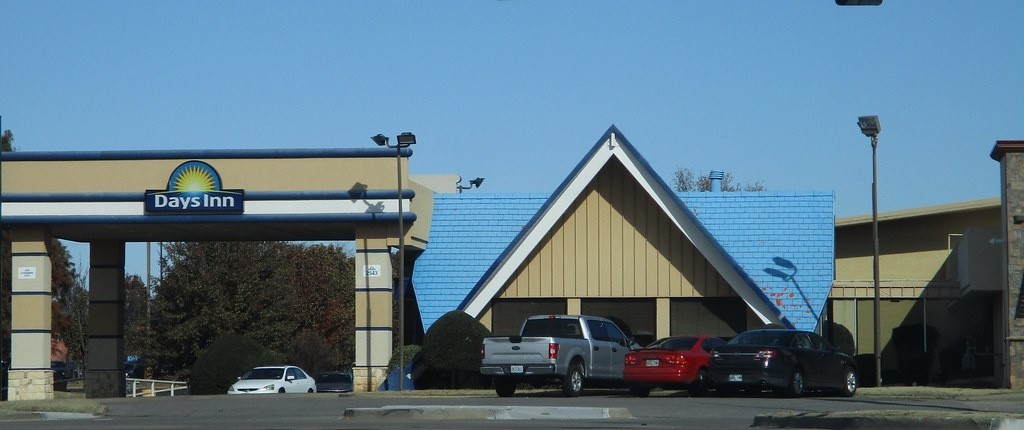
xmin=857 ymin=115 xmax=882 ymax=388
xmin=371 ymin=131 xmax=416 ymax=392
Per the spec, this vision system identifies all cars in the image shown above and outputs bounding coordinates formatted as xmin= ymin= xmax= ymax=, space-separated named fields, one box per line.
xmin=227 ymin=366 xmax=317 ymax=395
xmin=623 ymin=336 xmax=728 ymax=398
xmin=316 ymin=371 xmax=353 ymax=392
xmin=710 ymin=329 xmax=858 ymax=397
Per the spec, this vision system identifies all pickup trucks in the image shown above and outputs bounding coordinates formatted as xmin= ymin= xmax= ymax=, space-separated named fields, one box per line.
xmin=480 ymin=315 xmax=644 ymax=397
